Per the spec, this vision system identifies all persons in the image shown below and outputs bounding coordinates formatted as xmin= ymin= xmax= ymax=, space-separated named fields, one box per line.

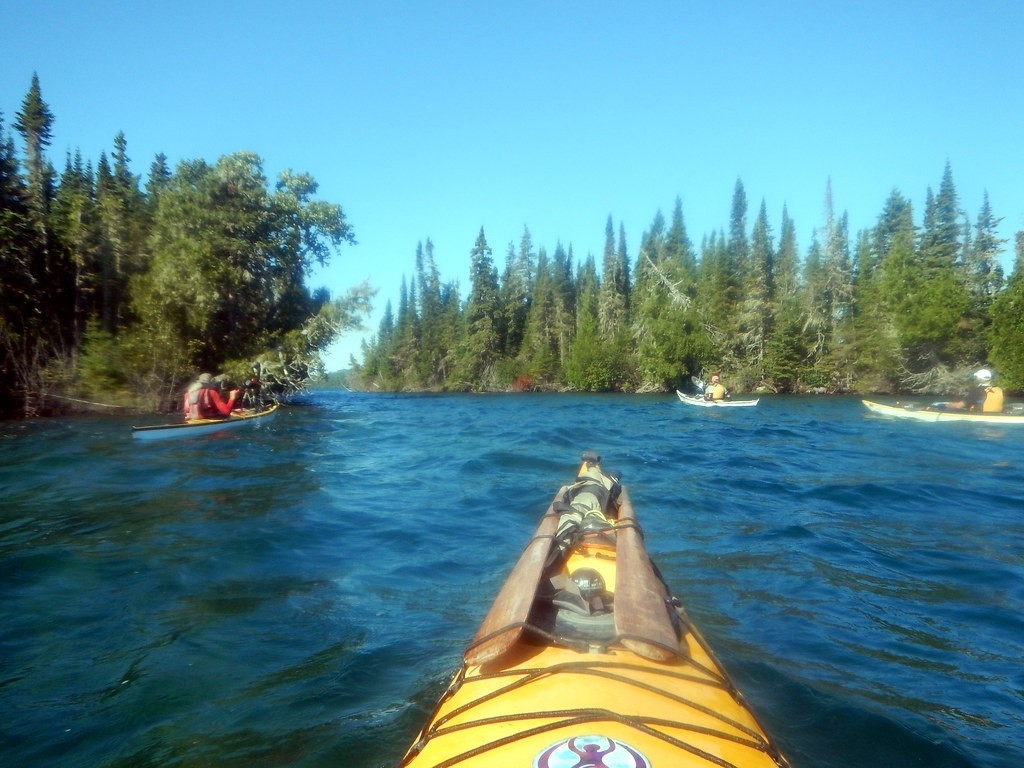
xmin=704 ymin=376 xmax=728 ymax=401
xmin=185 ymin=373 xmax=239 ymax=421
xmin=930 ymin=369 xmax=1004 ymax=412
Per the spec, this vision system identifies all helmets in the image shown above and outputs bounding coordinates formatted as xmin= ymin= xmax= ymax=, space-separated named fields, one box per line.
xmin=198 ymin=373 xmax=215 ymax=385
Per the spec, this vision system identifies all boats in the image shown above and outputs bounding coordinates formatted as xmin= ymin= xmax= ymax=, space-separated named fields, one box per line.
xmin=675 ymin=390 xmax=759 ymax=407
xmin=861 ymin=399 xmax=1024 ymax=425
xmin=132 ymin=399 xmax=278 ymax=442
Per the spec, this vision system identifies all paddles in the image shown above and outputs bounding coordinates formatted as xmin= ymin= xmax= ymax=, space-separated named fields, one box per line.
xmin=460 ymin=483 xmax=572 ymax=664
xmin=616 ymin=482 xmax=684 ymax=662
xmin=691 ymin=375 xmax=704 ymax=389
xmin=931 ymin=400 xmax=1024 ymax=414
